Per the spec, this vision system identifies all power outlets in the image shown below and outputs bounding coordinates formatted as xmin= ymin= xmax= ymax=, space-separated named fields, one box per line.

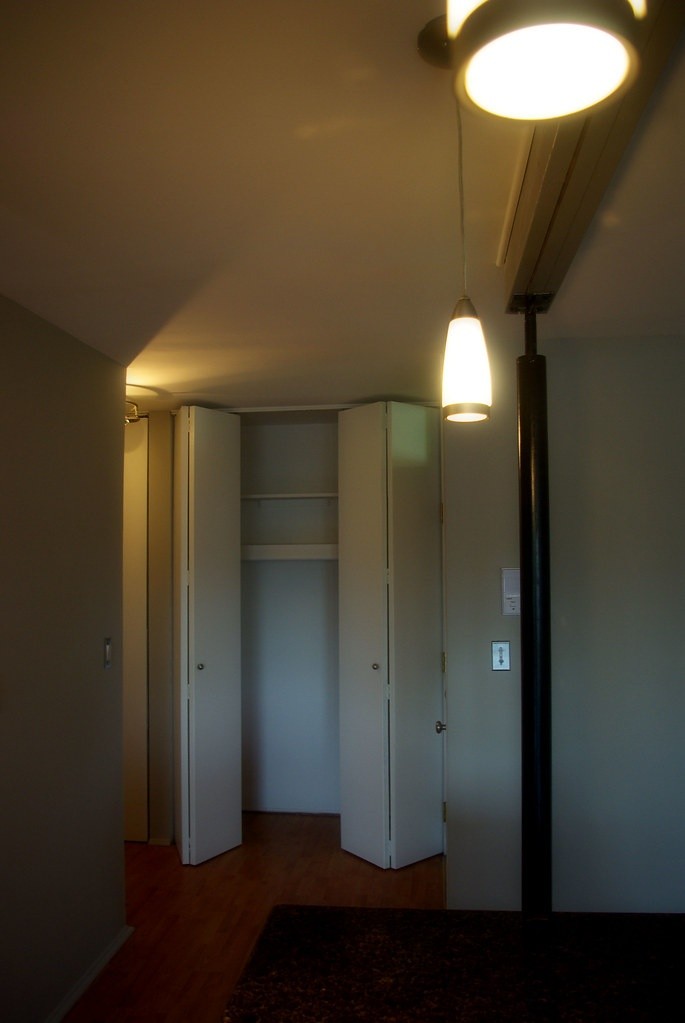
xmin=491 ymin=642 xmax=511 ymax=671
xmin=102 ymin=638 xmax=111 ymax=670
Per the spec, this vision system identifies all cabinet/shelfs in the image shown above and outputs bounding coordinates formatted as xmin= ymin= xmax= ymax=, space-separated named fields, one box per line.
xmin=169 ymin=401 xmax=445 ymax=869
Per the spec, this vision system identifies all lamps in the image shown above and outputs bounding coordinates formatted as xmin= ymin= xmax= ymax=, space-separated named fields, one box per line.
xmin=445 ymin=0 xmax=660 ymax=126
xmin=416 ymin=14 xmax=492 ymax=422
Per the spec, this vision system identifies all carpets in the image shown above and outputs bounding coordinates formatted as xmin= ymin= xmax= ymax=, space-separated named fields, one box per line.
xmin=220 ymin=904 xmax=685 ymax=1023
